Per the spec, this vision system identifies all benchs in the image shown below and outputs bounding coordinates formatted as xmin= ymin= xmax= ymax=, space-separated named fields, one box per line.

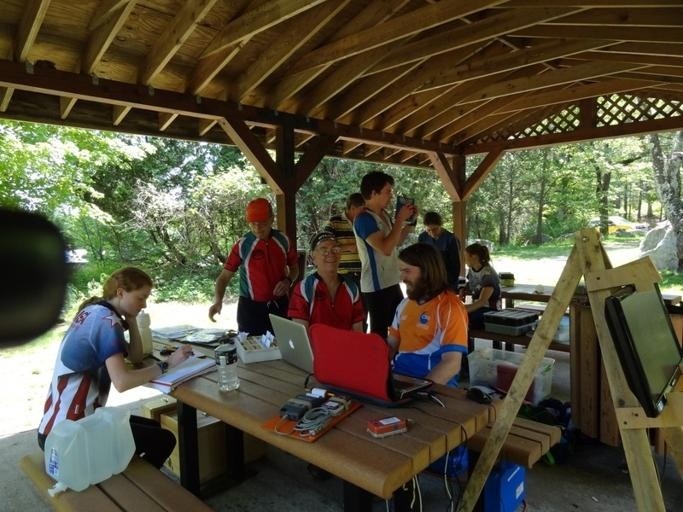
xmin=20 ymin=450 xmax=211 ymax=511
xmin=470 ymin=415 xmax=562 ymax=472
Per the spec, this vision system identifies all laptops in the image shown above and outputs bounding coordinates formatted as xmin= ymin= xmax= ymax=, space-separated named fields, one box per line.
xmin=268 ymin=312 xmax=319 ymax=376
xmin=308 ymin=324 xmax=434 ymax=399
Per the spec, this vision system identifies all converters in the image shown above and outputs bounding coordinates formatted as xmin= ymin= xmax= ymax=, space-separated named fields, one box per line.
xmin=366 ymin=415 xmax=409 ymax=438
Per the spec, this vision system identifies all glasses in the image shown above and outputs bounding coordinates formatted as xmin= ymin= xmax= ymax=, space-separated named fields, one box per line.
xmin=315 ymin=247 xmax=341 ymax=256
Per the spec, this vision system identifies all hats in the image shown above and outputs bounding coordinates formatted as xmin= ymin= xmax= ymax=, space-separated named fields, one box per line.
xmin=245 ymin=198 xmax=272 ymax=222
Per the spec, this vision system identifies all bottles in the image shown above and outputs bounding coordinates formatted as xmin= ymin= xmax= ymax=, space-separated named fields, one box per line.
xmin=213 ymin=338 xmax=240 ymax=393
xmin=132 ymin=310 xmax=151 ymax=355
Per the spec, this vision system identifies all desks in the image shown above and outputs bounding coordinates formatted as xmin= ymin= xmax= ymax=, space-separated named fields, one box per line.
xmin=458 ymin=283 xmax=569 ymax=381
xmin=123 ymin=324 xmax=507 ymax=510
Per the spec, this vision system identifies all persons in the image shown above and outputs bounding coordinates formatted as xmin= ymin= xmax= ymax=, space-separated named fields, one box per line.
xmin=463 ymin=243 xmax=502 ymax=313
xmin=323 ymin=193 xmax=369 ymax=333
xmin=382 ymin=241 xmax=469 ymax=388
xmin=418 ymin=211 xmax=461 ymax=293
xmin=37 ymin=266 xmax=194 ymax=472
xmin=209 ymin=197 xmax=299 ymax=336
xmin=287 ymin=230 xmax=366 ymax=332
xmin=353 ymin=170 xmax=420 ymax=336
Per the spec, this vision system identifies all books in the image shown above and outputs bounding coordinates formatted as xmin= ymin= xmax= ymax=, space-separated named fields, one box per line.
xmin=145 ymin=355 xmax=218 ymax=394
